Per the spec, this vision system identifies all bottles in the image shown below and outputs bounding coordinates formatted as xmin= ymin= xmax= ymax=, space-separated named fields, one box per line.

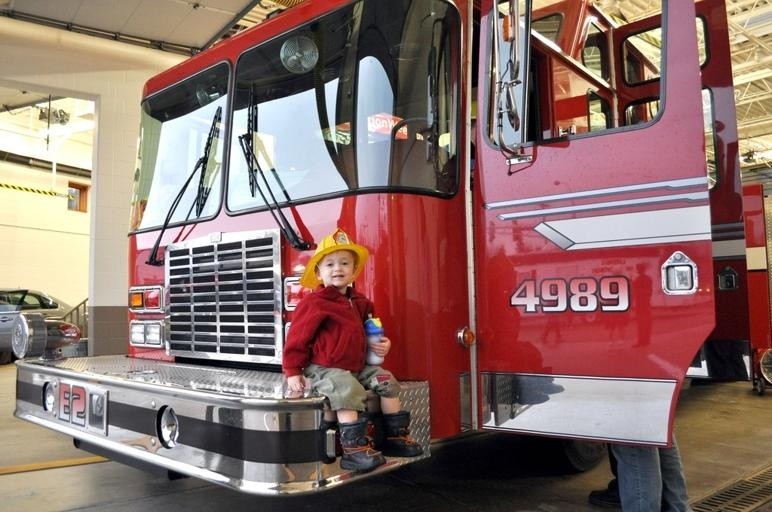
xmin=362 ymin=317 xmax=386 ymax=366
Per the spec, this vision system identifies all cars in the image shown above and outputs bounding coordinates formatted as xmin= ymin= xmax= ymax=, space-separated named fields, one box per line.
xmin=0 ymin=289 xmax=88 ymax=352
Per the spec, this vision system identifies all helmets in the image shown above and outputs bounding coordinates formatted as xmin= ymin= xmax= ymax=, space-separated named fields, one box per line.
xmin=299 ymin=229 xmax=369 ymax=290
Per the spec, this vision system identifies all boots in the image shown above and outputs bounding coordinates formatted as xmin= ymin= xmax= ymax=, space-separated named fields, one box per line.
xmin=338 ymin=417 xmax=386 ymax=471
xmin=373 ymin=412 xmax=424 ymax=458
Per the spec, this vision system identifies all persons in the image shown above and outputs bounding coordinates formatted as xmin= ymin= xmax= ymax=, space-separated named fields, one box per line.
xmin=281 ymin=228 xmax=424 ymax=470
xmin=611 ymin=428 xmax=691 ymax=511
xmin=587 ymin=441 xmax=621 ymax=508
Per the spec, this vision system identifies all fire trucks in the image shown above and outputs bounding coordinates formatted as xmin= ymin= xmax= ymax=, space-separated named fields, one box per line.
xmin=12 ymin=0 xmax=772 ymax=496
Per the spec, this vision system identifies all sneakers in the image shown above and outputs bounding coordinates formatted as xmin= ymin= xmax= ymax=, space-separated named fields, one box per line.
xmin=588 ymin=488 xmax=623 ymax=508
